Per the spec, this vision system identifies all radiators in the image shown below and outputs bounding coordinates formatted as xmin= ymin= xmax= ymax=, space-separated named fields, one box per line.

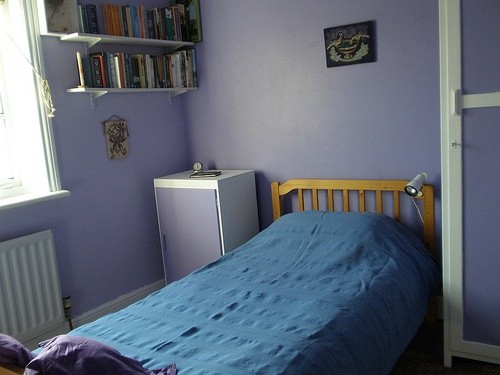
xmin=0 ymin=229 xmax=65 ymax=344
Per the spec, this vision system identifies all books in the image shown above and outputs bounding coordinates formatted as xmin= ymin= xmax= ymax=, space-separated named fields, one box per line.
xmin=78 ymin=0 xmax=202 ymax=42
xmin=77 ymin=47 xmax=197 ymax=88
xmin=190 ymin=171 xmax=221 ymax=177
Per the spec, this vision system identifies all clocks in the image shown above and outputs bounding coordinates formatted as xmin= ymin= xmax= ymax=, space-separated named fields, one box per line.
xmin=193 ymin=163 xmax=201 ymax=171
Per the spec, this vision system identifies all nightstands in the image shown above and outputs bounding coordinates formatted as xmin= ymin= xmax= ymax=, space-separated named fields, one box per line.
xmin=154 ymin=170 xmax=259 ymax=287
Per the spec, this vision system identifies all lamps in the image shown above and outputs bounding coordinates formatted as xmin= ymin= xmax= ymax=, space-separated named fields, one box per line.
xmin=404 ymin=172 xmax=426 ymax=197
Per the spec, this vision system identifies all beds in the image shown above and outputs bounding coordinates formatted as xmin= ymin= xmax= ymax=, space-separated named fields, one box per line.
xmin=0 ymin=179 xmax=435 ymax=375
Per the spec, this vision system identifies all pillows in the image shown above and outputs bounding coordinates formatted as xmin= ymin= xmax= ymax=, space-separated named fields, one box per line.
xmin=23 ymin=336 xmax=177 ymax=375
xmin=0 ymin=333 xmax=39 ymax=367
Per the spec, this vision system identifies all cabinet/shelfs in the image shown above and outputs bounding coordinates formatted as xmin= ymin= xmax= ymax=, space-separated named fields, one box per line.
xmin=439 ymin=0 xmax=500 ymax=368
xmin=37 ymin=0 xmax=198 ymax=111
